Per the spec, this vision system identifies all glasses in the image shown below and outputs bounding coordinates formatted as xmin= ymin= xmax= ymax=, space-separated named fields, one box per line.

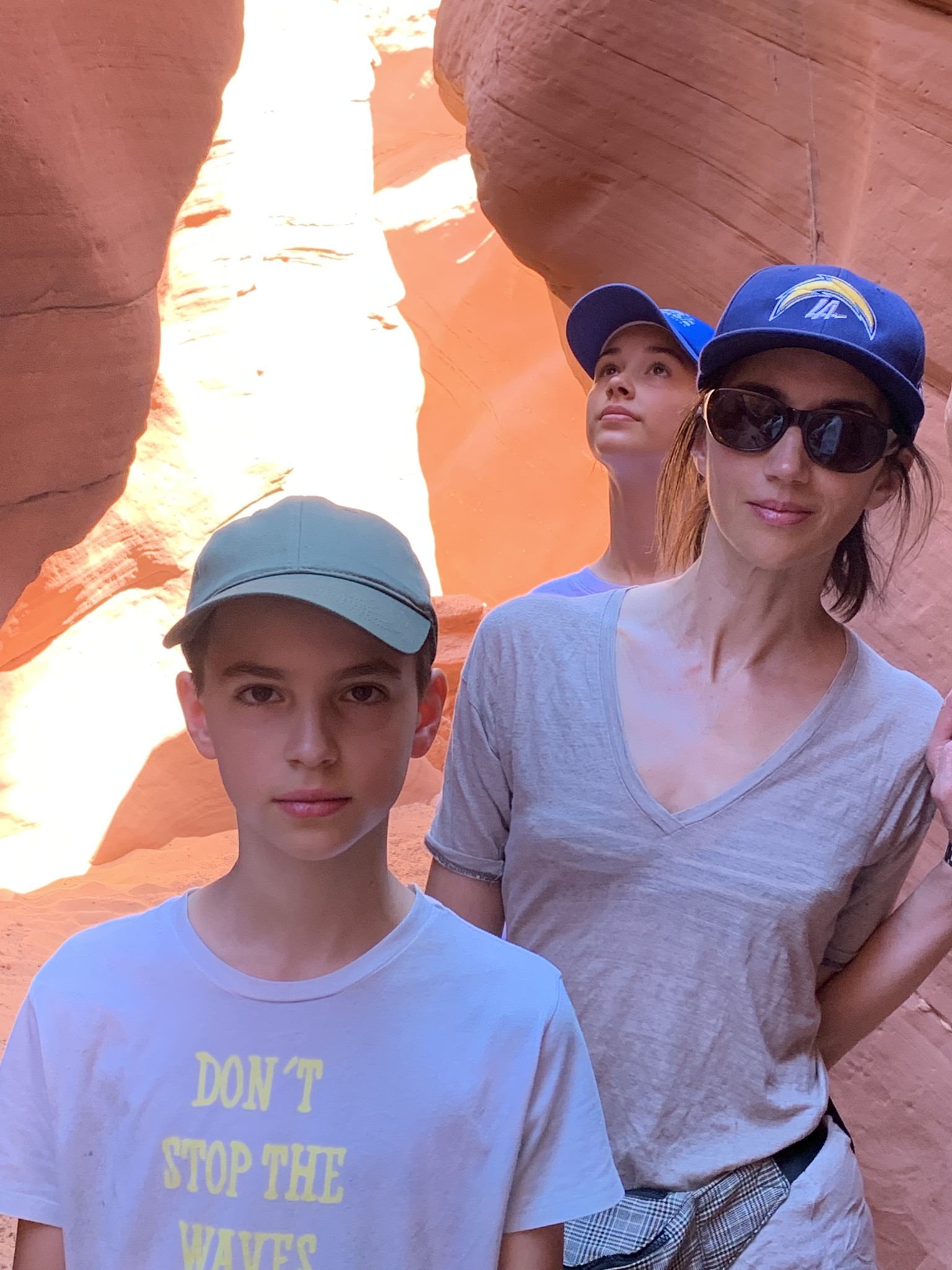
xmin=700 ymin=385 xmax=904 ymax=472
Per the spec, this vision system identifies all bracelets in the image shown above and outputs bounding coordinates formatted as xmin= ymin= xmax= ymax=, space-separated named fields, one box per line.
xmin=944 ymin=840 xmax=952 ymax=867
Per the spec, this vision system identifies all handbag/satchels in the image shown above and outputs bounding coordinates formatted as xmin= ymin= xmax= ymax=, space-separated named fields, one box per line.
xmin=563 ymin=1094 xmax=856 ymax=1270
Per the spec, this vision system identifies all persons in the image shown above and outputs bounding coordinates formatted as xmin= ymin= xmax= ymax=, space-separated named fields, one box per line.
xmin=532 ymin=283 xmax=715 ymax=599
xmin=0 ymin=499 xmax=623 ymax=1270
xmin=424 ymin=264 xmax=952 ymax=1270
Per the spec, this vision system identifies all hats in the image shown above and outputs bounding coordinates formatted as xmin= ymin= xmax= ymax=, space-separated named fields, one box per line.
xmin=162 ymin=495 xmax=439 ymax=659
xmin=564 ymin=282 xmax=714 ymax=382
xmin=698 ymin=264 xmax=925 ymax=424
